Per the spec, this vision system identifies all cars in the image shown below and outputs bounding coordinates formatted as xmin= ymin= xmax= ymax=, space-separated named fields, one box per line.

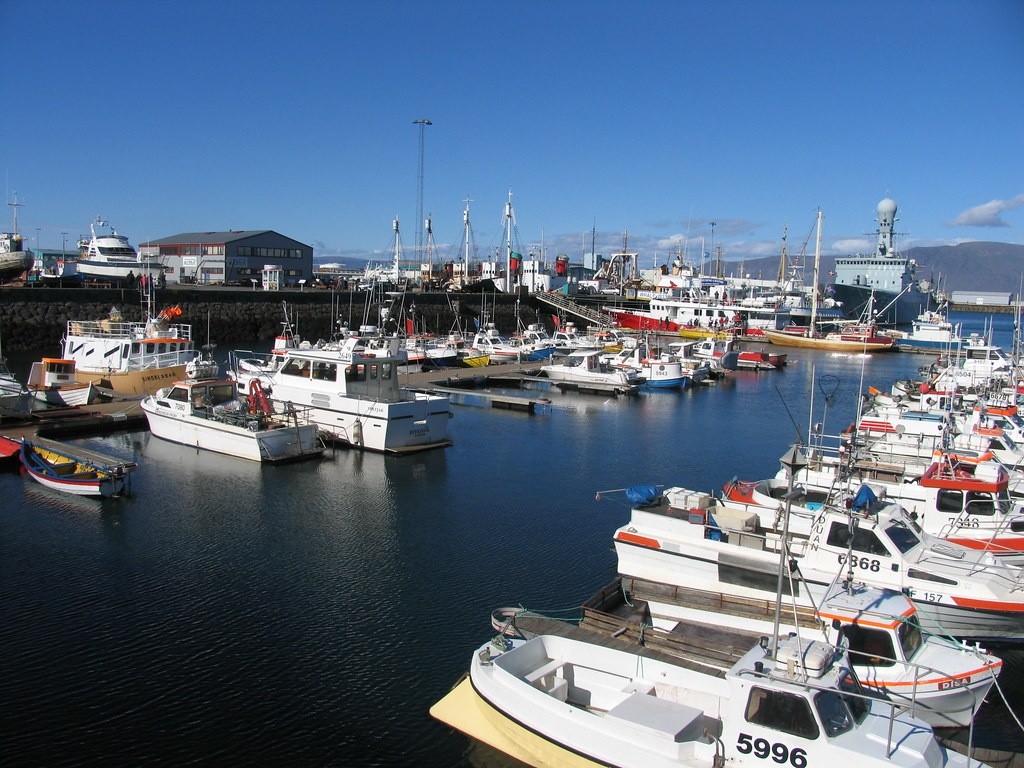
xmin=577 ymin=284 xmax=597 ymax=295
xmin=598 ymin=286 xmax=619 ymax=295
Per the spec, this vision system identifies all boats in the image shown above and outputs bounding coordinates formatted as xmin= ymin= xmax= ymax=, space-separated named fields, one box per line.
xmin=576 ymin=501 xmax=1003 ymax=729
xmin=614 ymin=325 xmax=1024 ymax=643
xmin=26 ymin=242 xmax=203 ymax=408
xmin=0 ymin=352 xmax=99 ymax=421
xmin=226 ymin=332 xmax=455 ymax=456
xmin=140 ymin=311 xmax=328 ymax=465
xmin=21 ymin=434 xmax=125 ymax=500
xmin=826 ymin=197 xmax=954 ymax=327
xmin=0 ymin=233 xmax=35 ymax=285
xmin=41 ymin=261 xmax=81 ymax=288
xmin=271 ymin=272 xmax=788 ymax=396
xmin=876 ymin=272 xmax=986 ymax=351
xmin=720 ymin=322 xmax=1024 ymax=566
xmin=774 ymin=292 xmax=1024 ymax=501
xmin=75 ymin=214 xmax=170 ymax=288
xmin=428 ymin=364 xmax=993 ymax=768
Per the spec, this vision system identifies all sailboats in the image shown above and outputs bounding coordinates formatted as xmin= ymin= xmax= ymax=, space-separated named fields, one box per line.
xmin=761 ymin=212 xmax=896 ymax=352
xmin=677 ymin=293 xmax=727 ymax=340
xmin=602 ymin=220 xmax=844 ymax=336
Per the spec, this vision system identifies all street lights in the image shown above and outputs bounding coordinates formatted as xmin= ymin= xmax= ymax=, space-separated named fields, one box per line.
xmin=36 ymin=228 xmax=41 ymax=271
xmin=61 ymin=233 xmax=68 ymax=261
